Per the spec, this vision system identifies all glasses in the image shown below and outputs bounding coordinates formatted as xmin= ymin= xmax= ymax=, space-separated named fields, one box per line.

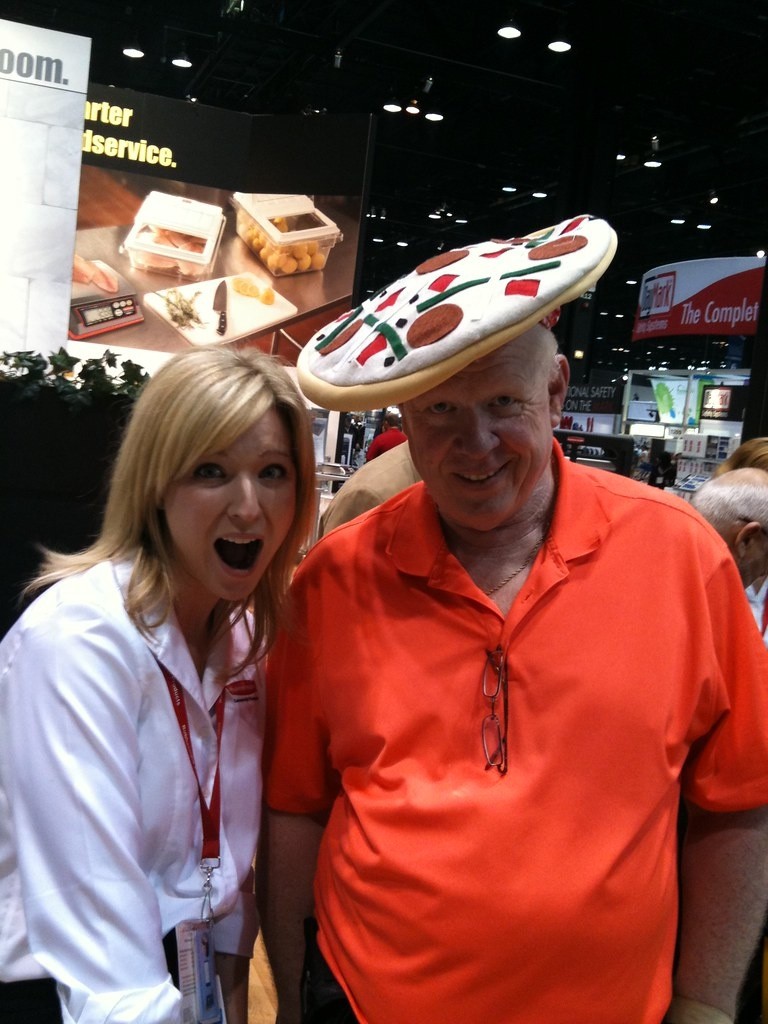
xmin=482 ymin=643 xmax=509 ymax=775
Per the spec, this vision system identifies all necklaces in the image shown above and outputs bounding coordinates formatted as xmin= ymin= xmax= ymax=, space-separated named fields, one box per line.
xmin=484 ymin=519 xmax=550 ymax=596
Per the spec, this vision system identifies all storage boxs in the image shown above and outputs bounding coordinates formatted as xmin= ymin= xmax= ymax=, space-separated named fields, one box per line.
xmin=124 ymin=189 xmax=227 ymax=280
xmin=227 ymin=192 xmax=344 ymax=277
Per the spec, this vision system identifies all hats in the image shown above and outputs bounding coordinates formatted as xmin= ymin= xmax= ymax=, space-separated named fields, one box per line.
xmin=296 ymin=214 xmax=618 ymax=412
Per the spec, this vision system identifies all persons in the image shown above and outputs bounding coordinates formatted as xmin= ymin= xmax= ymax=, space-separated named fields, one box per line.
xmin=689 ymin=435 xmax=768 ymax=642
xmin=257 ymin=216 xmax=768 ymax=1024
xmin=0 ymin=351 xmax=317 ymax=1024
xmin=318 ymin=413 xmax=422 ymax=542
